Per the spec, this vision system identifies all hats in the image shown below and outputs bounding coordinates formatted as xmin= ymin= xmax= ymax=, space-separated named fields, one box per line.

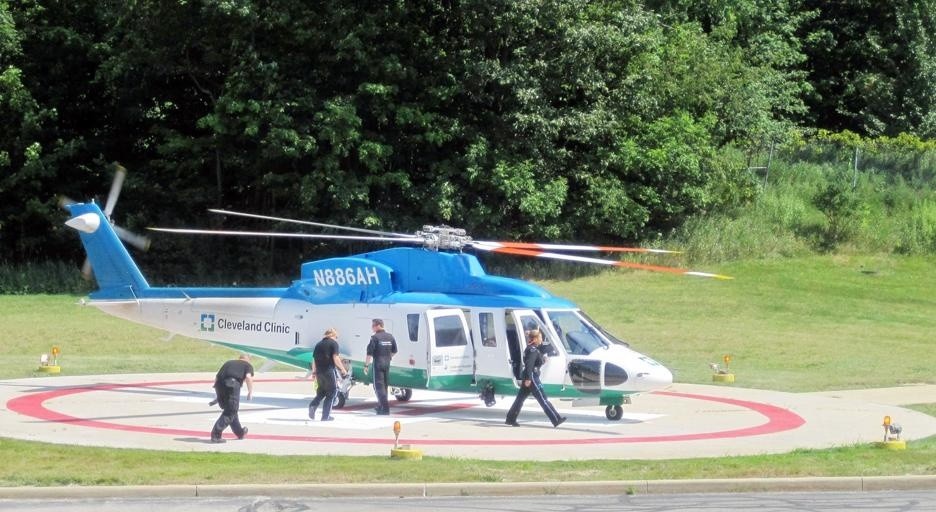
xmin=325 ymin=328 xmax=337 ymax=337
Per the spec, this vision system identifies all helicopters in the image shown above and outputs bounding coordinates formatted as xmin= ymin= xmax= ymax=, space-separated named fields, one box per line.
xmin=55 ymin=158 xmax=736 ymax=424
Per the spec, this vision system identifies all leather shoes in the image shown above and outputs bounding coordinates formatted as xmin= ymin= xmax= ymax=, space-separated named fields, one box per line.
xmin=321 ymin=416 xmax=333 ymax=421
xmin=377 ymin=411 xmax=388 ymax=415
xmin=506 ymin=419 xmax=520 ymax=427
xmin=211 ymin=438 xmax=225 ymax=443
xmin=554 ymin=417 xmax=566 ymax=427
xmin=375 ymin=407 xmax=383 ymax=411
xmin=308 ymin=406 xmax=314 ymax=419
xmin=238 ymin=427 xmax=247 ymax=439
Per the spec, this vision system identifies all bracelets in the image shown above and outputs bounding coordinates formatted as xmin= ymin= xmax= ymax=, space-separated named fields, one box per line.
xmin=364 ymin=364 xmax=369 ymax=368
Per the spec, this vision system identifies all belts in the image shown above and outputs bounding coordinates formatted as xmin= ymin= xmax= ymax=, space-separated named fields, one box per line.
xmin=225 ymin=376 xmax=239 ymax=382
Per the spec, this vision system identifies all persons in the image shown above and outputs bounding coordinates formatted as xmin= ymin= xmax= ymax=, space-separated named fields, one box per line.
xmin=210 ymin=352 xmax=255 ymax=444
xmin=505 ymin=330 xmax=567 ymax=428
xmin=305 ymin=329 xmax=347 ymax=422
xmin=363 ymin=317 xmax=398 ymax=415
